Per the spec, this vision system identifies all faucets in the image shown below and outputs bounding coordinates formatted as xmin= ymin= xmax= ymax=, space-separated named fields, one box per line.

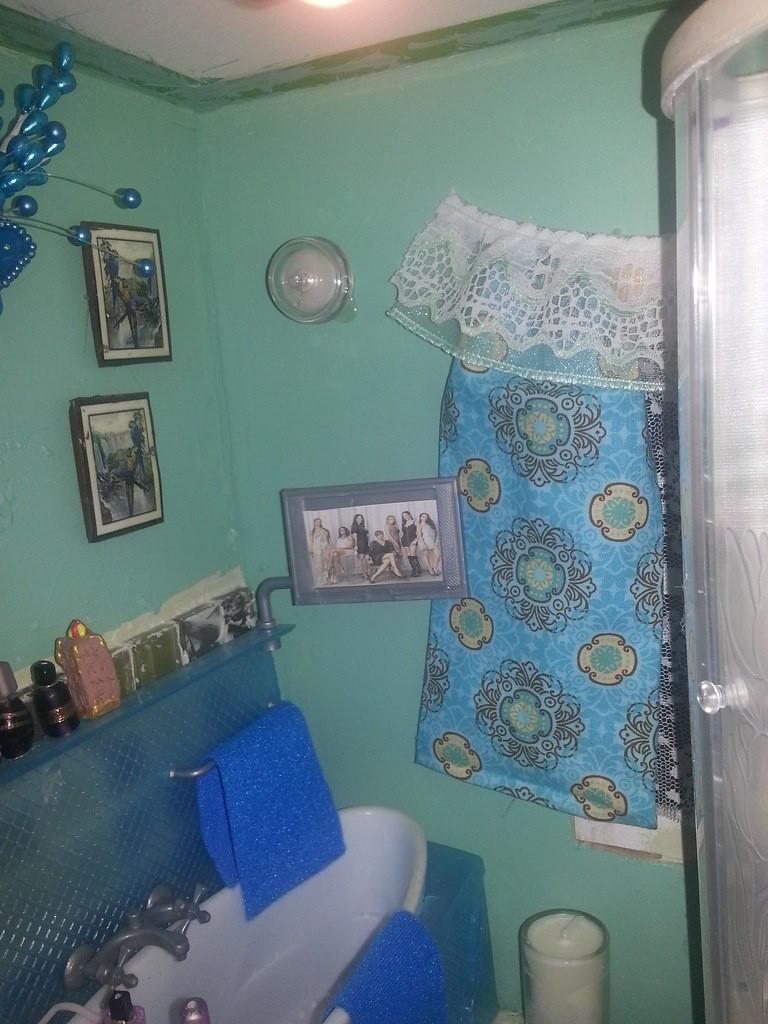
xmin=65 ymin=883 xmax=221 ymax=996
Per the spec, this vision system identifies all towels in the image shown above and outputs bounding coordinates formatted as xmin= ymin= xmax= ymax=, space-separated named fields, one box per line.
xmin=333 ymin=907 xmax=447 ymax=1024
xmin=200 ymin=700 xmax=347 ymax=923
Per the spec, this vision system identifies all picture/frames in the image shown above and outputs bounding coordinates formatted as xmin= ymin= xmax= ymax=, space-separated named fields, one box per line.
xmin=78 ymin=220 xmax=173 ymax=367
xmin=69 ymin=392 xmax=164 ymax=544
xmin=279 ymin=475 xmax=472 ymax=608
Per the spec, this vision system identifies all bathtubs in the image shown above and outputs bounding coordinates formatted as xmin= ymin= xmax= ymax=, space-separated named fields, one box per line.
xmin=64 ymin=804 xmax=427 ymax=1023
